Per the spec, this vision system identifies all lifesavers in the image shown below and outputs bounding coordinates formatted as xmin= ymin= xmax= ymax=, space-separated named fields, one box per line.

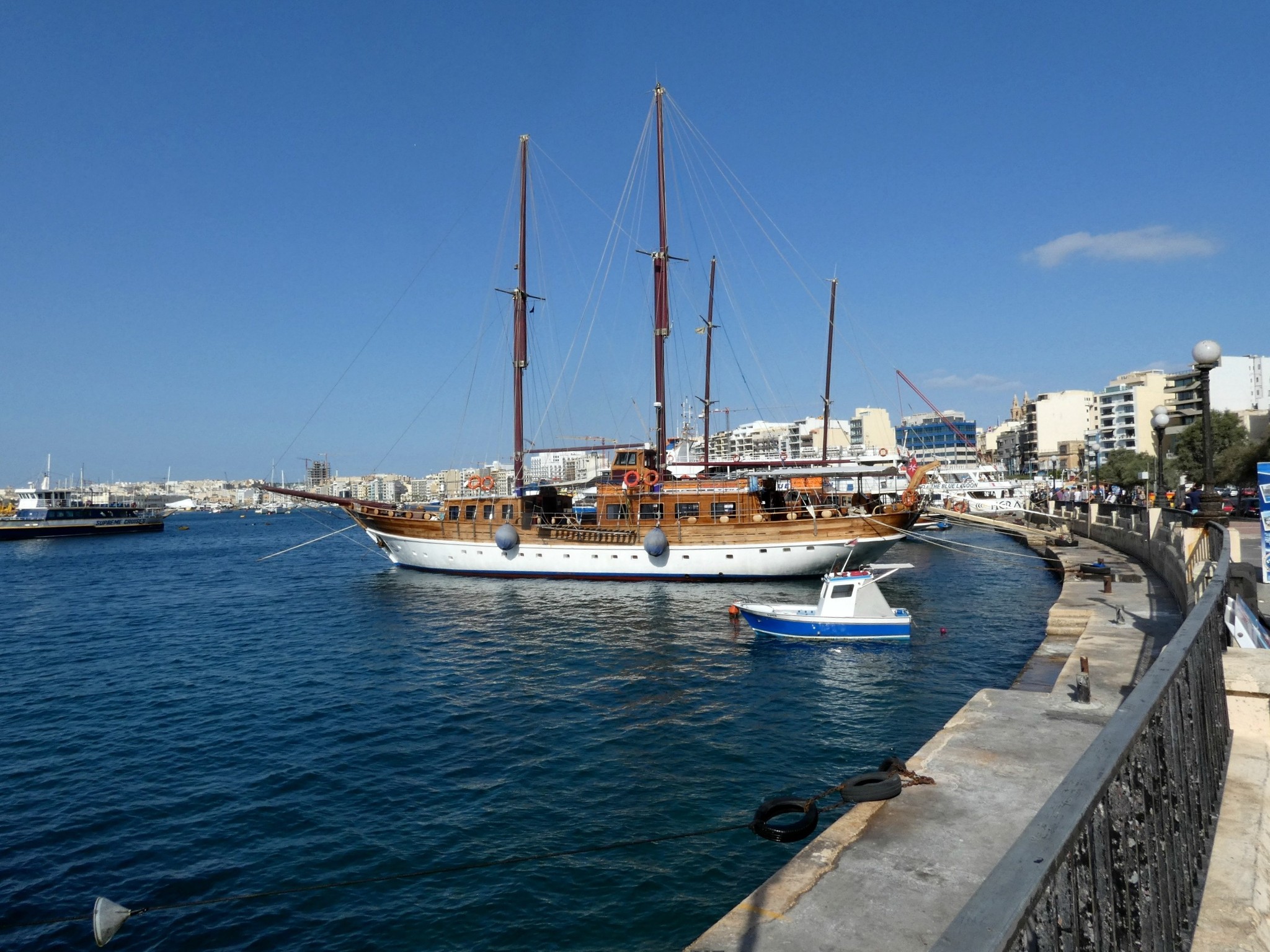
xmin=469 ymin=476 xmax=481 ymax=489
xmin=879 ymin=448 xmax=887 ymax=456
xmin=754 ymin=797 xmax=818 ymax=842
xmin=780 ymin=453 xmax=787 ymax=460
xmin=954 ymin=502 xmax=966 ymax=513
xmin=915 ymin=491 xmax=920 ymax=504
xmin=841 ymin=772 xmax=901 ymax=800
xmin=680 ymin=475 xmax=705 ymax=477
xmin=902 ymin=490 xmax=914 ymax=507
xmin=907 ymin=451 xmax=909 ymax=456
xmin=1055 ymin=539 xmax=1078 ymax=547
xmin=667 ymin=455 xmax=673 ymax=462
xmin=898 ymin=449 xmax=900 ymax=454
xmin=481 ymin=477 xmax=493 ymax=490
xmin=699 ymin=456 xmax=705 ymax=462
xmin=1080 ymin=564 xmax=1110 ymax=574
xmin=733 ymin=455 xmax=740 ymax=462
xmin=624 ymin=470 xmax=640 ymax=487
xmin=644 ymin=470 xmax=659 ymax=486
xmin=842 ymin=571 xmax=868 ymax=576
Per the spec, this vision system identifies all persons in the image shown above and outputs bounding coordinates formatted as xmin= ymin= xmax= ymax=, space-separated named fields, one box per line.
xmin=1184 ymin=487 xmax=1201 ymax=515
xmin=1030 ymin=485 xmax=1156 ymax=508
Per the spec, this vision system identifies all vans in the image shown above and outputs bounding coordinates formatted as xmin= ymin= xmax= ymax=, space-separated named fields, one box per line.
xmin=1171 ymin=484 xmax=1204 ymax=509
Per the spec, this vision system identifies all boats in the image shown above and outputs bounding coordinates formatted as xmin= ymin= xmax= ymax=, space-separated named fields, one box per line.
xmin=725 ymin=538 xmax=922 ymax=644
xmin=893 ymin=370 xmax=1030 ymax=531
xmin=211 ymin=506 xmax=232 ymax=515
xmin=0 ymin=452 xmax=178 ymax=539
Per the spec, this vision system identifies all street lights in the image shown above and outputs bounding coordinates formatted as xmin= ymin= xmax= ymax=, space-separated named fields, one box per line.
xmin=1189 ymin=338 xmax=1230 ymax=527
xmin=1090 ymin=443 xmax=1105 ymax=504
xmin=1152 ymin=404 xmax=1172 ymax=507
xmin=1051 ymin=455 xmax=1058 ymax=496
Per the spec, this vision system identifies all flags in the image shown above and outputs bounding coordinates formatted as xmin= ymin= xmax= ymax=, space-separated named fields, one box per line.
xmin=907 ymin=457 xmax=918 ymax=479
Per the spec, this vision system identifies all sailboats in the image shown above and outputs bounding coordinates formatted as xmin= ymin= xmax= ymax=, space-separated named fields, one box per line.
xmin=255 ymin=458 xmax=302 ymax=516
xmin=251 ymin=86 xmax=922 ymax=582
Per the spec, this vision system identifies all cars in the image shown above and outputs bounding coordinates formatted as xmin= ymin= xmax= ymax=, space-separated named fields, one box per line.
xmin=1231 ymin=497 xmax=1261 ymax=519
xmin=1221 ymin=499 xmax=1236 ymax=512
xmin=1241 ymin=488 xmax=1256 ymax=497
xmin=1215 ymin=487 xmax=1225 ymax=497
xmin=1222 ymin=486 xmax=1239 ymax=497
